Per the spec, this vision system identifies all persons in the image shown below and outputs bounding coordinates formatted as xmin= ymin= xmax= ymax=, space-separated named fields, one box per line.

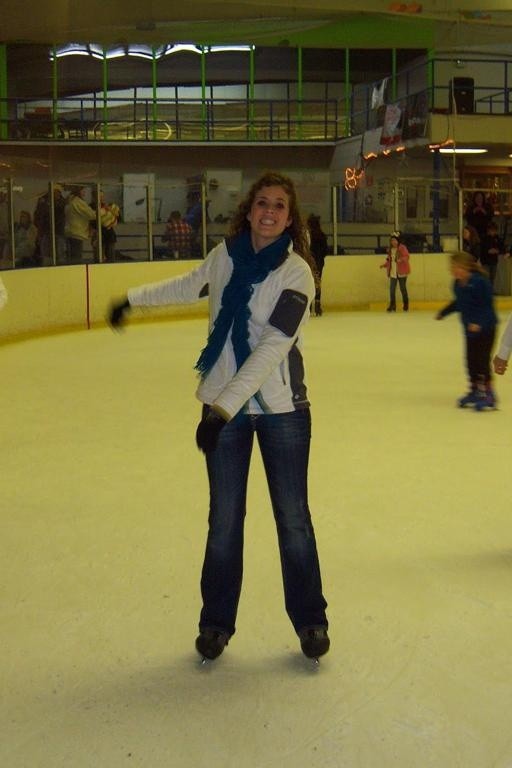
xmin=493 ymin=314 xmax=511 ymax=376
xmin=162 ymin=191 xmax=202 ymax=260
xmin=463 ymin=189 xmax=505 ymax=263
xmin=3 ymin=183 xmax=120 ymax=268
xmin=435 ymin=249 xmax=498 ymax=409
xmin=380 ymin=229 xmax=414 ymax=313
xmin=105 ymin=173 xmax=330 ymax=660
xmin=303 ymin=212 xmax=329 ymax=316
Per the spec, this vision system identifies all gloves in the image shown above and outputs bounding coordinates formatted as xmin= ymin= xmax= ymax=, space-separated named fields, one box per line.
xmin=197 ymin=414 xmax=225 ymax=453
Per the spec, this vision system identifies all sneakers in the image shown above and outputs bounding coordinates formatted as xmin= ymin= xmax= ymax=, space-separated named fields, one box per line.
xmin=195 ymin=628 xmax=228 ymax=660
xmin=460 ymin=389 xmax=495 ymax=410
xmin=299 ymin=628 xmax=329 ymax=657
